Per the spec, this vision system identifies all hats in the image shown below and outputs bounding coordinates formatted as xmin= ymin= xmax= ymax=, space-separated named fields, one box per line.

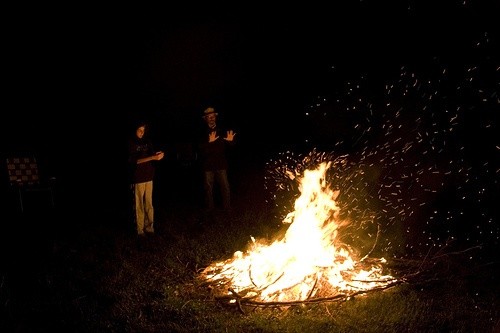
xmin=202 ymin=107 xmax=218 ymax=117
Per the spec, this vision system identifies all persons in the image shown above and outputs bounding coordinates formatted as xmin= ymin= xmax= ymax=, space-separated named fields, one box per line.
xmin=196 ymin=104 xmax=236 ymax=218
xmin=126 ymin=122 xmax=165 ymax=237
